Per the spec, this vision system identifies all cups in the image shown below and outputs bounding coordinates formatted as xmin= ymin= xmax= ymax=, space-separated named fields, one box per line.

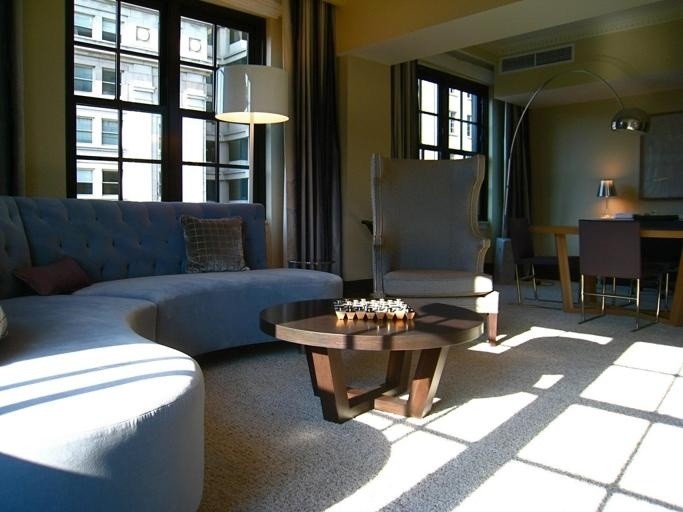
xmin=333 ymin=297 xmax=415 ymax=320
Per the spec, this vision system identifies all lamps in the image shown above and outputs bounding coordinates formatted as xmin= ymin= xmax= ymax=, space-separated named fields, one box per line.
xmin=214 ymin=65 xmax=289 ymax=203
xmin=597 ymin=180 xmax=617 ymax=218
xmin=495 ymin=68 xmax=651 ymax=286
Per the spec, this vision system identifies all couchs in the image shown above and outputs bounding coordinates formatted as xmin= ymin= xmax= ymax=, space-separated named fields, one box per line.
xmin=1 ymin=197 xmax=345 ymax=511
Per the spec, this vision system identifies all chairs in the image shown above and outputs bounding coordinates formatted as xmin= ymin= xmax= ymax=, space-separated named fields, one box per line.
xmin=579 ymin=220 xmax=661 ymax=332
xmin=371 ymin=156 xmax=500 ymax=345
xmin=507 ymin=217 xmax=561 ymax=305
xmin=632 ymin=214 xmax=681 ymax=315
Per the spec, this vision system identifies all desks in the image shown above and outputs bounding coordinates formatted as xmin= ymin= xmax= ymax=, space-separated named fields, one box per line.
xmin=527 ymin=224 xmax=683 ymax=328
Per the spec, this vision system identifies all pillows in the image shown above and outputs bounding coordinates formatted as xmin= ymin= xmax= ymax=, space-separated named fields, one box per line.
xmin=13 ymin=258 xmax=91 ymax=298
xmin=180 ymin=216 xmax=251 ymax=273
